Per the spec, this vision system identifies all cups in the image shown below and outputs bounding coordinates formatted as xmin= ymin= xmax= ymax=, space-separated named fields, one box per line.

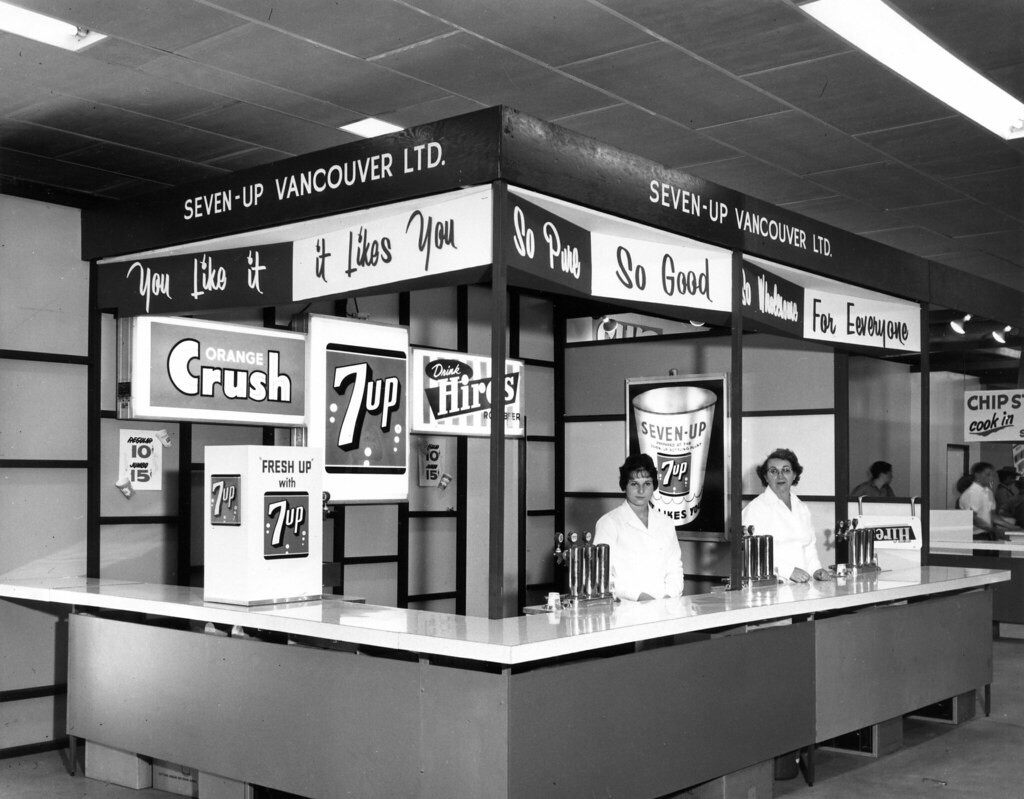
xmin=630 ymin=386 xmax=717 ymax=526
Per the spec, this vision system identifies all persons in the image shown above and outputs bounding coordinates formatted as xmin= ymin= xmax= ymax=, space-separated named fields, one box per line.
xmin=956 ymin=465 xmax=1024 ymax=528
xmin=583 ymin=453 xmax=684 ymax=600
xmin=958 ymin=462 xmax=1021 ymax=557
xmin=853 ymin=461 xmax=896 ymax=498
xmin=741 ymin=449 xmax=832 ymax=583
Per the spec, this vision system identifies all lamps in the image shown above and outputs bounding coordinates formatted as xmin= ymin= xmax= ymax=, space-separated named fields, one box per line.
xmin=602 ymin=315 xmax=618 ymax=332
xmin=690 ymin=320 xmax=705 ymax=327
xmin=992 ymin=325 xmax=1012 ymax=343
xmin=950 ymin=314 xmax=974 ymax=335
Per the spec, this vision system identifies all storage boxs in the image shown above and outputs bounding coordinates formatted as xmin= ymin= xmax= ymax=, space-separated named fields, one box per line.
xmin=152 ymin=757 xmax=199 ymax=797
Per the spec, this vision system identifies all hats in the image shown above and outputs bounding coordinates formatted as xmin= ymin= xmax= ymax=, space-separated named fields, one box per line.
xmin=997 ymin=466 xmax=1022 ymax=476
